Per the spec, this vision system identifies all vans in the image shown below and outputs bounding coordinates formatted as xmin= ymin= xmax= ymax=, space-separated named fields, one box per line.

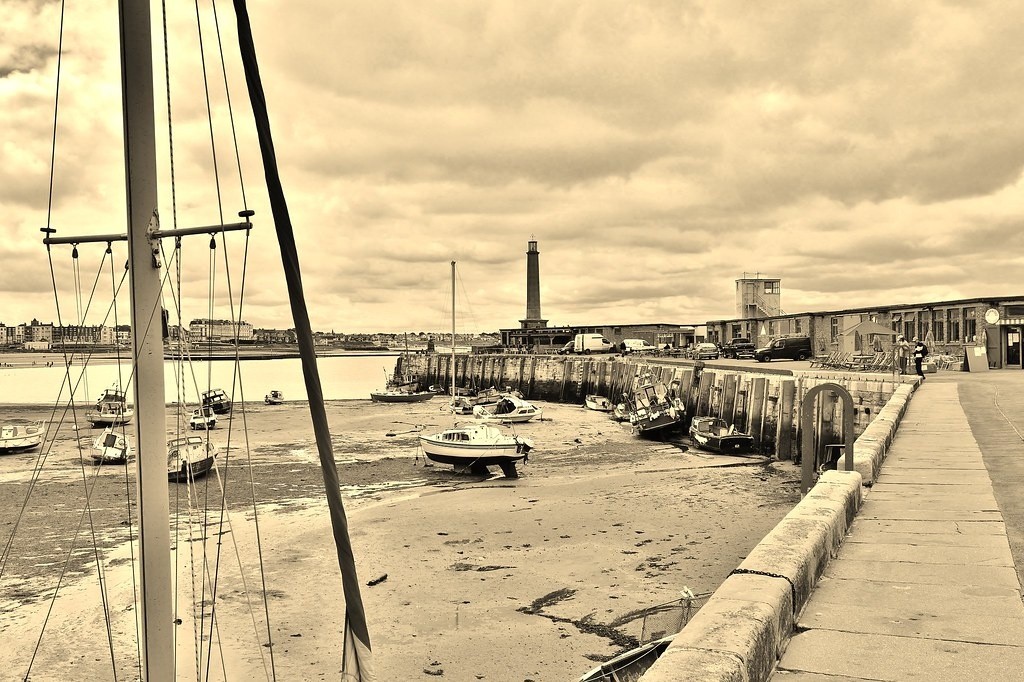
xmin=621 ymin=338 xmax=657 ymax=354
xmin=752 ymin=337 xmax=813 ymax=362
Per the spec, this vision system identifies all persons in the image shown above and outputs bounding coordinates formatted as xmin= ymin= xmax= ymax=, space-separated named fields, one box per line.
xmin=911 ymin=337 xmax=929 ymax=379
xmin=896 ymin=334 xmax=909 ymax=375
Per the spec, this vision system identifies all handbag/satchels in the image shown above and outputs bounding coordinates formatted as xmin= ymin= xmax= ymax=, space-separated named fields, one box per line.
xmin=923 ymin=345 xmax=928 ymax=356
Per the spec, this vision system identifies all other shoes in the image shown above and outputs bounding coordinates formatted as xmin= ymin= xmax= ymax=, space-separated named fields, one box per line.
xmin=923 ymin=377 xmax=926 ymax=380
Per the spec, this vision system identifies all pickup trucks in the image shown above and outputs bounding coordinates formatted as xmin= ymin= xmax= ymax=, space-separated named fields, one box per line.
xmin=722 ymin=338 xmax=757 ymax=359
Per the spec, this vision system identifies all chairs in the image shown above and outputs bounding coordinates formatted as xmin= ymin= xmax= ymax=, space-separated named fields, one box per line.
xmin=810 ymin=351 xmax=964 ymax=372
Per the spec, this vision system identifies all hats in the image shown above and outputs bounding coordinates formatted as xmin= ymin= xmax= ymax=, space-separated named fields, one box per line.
xmin=897 ymin=335 xmax=903 ymax=341
xmin=912 ymin=337 xmax=918 ymax=341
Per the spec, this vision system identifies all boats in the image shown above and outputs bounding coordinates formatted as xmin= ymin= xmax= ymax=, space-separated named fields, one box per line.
xmin=167 ymin=436 xmax=218 ymax=483
xmin=449 ymin=384 xmax=544 ymax=424
xmin=585 ymin=394 xmax=616 ymax=412
xmin=369 ymin=333 xmax=437 ymax=403
xmin=264 ymin=391 xmax=284 ymax=405
xmin=91 ymin=425 xmax=131 ymax=465
xmin=688 ymin=415 xmax=754 ymax=451
xmin=0 ymin=418 xmax=47 ymax=454
xmin=382 ymin=366 xmax=419 ymax=394
xmin=85 ymin=379 xmax=133 ymax=427
xmin=429 ymin=384 xmax=444 ymax=395
xmin=614 ymin=351 xmax=686 ymax=433
xmin=190 ymin=407 xmax=215 ymax=429
xmin=194 ymin=388 xmax=232 ymax=414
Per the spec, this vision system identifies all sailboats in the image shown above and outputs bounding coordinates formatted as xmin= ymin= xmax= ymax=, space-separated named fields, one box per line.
xmin=0 ymin=0 xmax=681 ymax=682
xmin=418 ymin=258 xmax=536 ymax=464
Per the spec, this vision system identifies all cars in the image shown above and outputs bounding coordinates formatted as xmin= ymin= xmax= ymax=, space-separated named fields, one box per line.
xmin=692 ymin=342 xmax=719 ymax=360
xmin=557 ymin=340 xmax=574 ymax=354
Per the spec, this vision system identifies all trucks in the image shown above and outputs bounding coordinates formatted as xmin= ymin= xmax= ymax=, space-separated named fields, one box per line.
xmin=574 ymin=333 xmax=614 ymax=355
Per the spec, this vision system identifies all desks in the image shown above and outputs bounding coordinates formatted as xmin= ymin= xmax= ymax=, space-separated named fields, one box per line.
xmin=954 ymin=355 xmax=964 ymax=371
xmin=849 ymin=355 xmax=874 ymax=372
xmin=810 ymin=354 xmax=828 ymax=368
